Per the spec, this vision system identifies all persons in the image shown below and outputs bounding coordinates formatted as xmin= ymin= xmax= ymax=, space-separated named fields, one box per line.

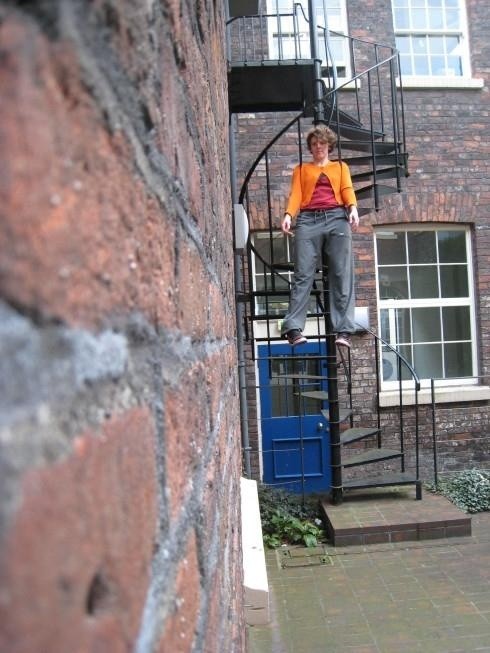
xmin=281 ymin=124 xmax=360 ymax=348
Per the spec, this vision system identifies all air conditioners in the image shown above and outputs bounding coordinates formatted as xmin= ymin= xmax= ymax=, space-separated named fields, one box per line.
xmin=382 ymin=351 xmax=398 ymax=381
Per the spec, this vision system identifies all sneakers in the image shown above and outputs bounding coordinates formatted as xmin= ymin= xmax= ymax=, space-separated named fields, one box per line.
xmin=335 ymin=332 xmax=352 ymax=348
xmin=286 ymin=329 xmax=307 ymax=346
